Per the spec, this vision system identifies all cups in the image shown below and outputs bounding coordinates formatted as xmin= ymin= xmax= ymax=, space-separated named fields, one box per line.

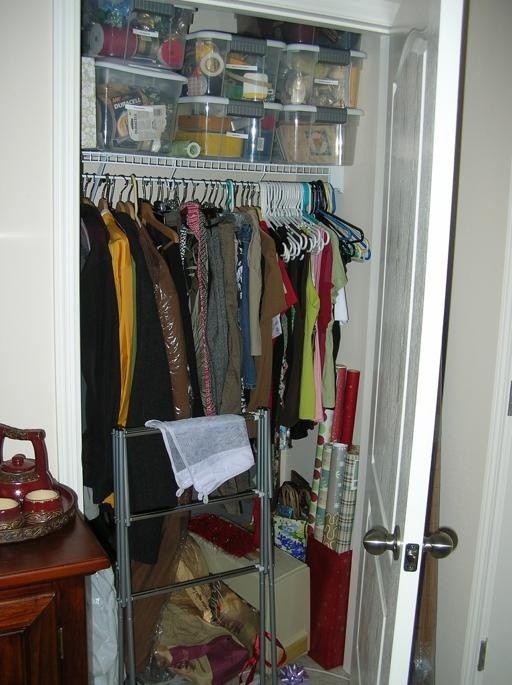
xmin=0 ymin=498 xmax=25 ymax=532
xmin=22 ymin=488 xmax=64 ymax=528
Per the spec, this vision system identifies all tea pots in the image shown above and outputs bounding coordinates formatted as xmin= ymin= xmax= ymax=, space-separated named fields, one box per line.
xmin=0 ymin=422 xmax=55 ymax=510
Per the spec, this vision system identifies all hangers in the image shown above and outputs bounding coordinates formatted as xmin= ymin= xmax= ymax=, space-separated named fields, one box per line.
xmin=82 ymin=179 xmax=378 ymax=269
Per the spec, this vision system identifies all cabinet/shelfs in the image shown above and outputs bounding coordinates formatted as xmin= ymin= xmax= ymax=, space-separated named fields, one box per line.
xmin=0 ymin=574 xmax=110 ymax=685
